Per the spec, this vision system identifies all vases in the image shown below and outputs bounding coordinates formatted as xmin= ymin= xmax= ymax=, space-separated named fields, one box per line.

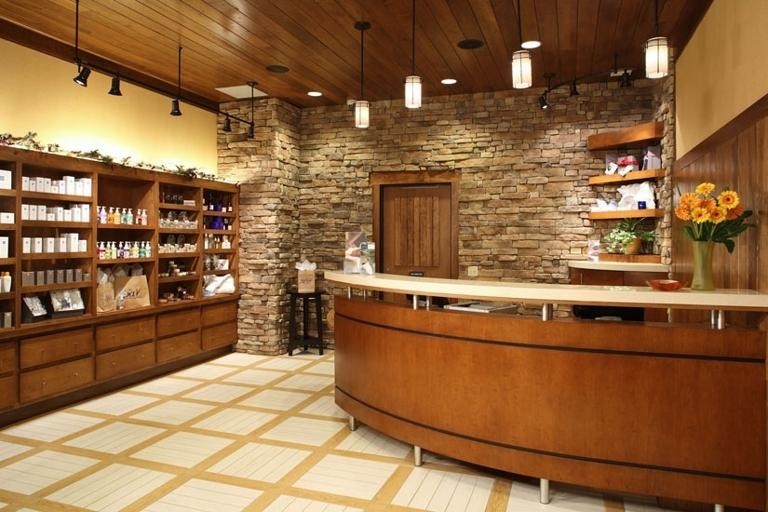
xmin=690 ymin=240 xmax=715 ymax=291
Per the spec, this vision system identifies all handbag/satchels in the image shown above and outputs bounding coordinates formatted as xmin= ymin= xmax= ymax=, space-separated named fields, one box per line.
xmin=96 ymin=280 xmax=118 ymax=313
xmin=115 ymin=273 xmax=151 ymax=311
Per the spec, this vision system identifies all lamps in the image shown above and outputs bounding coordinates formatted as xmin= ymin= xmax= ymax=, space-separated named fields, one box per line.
xmin=539 ymin=54 xmax=633 ymax=111
xmin=511 ymin=1 xmax=533 ymax=89
xmin=72 ymin=1 xmax=254 ymax=138
xmin=642 ymin=1 xmax=670 ymax=80
xmin=355 ymin=20 xmax=371 ymax=130
xmin=404 ymin=1 xmax=422 ymax=109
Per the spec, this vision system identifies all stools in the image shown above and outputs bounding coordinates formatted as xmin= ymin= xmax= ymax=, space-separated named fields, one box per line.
xmin=287 ymin=289 xmax=326 ymax=357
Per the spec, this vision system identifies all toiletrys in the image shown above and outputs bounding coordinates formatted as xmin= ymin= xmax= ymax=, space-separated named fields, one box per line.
xmin=1 ymin=272 xmax=12 ymax=293
xmin=97 ymin=205 xmax=153 ymax=260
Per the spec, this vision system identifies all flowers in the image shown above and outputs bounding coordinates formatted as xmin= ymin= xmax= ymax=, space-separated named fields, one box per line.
xmin=674 ymin=181 xmax=758 ymax=254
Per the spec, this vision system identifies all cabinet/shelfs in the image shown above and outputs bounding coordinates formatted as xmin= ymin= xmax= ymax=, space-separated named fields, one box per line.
xmin=587 ymin=122 xmax=664 ymax=264
xmin=0 ymin=145 xmax=240 ymax=431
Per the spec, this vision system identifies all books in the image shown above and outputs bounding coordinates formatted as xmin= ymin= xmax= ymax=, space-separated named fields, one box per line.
xmin=443 ymin=301 xmax=519 ymax=313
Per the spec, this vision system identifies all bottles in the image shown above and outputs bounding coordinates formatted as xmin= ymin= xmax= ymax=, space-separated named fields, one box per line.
xmin=0 ymin=272 xmax=12 ymax=293
